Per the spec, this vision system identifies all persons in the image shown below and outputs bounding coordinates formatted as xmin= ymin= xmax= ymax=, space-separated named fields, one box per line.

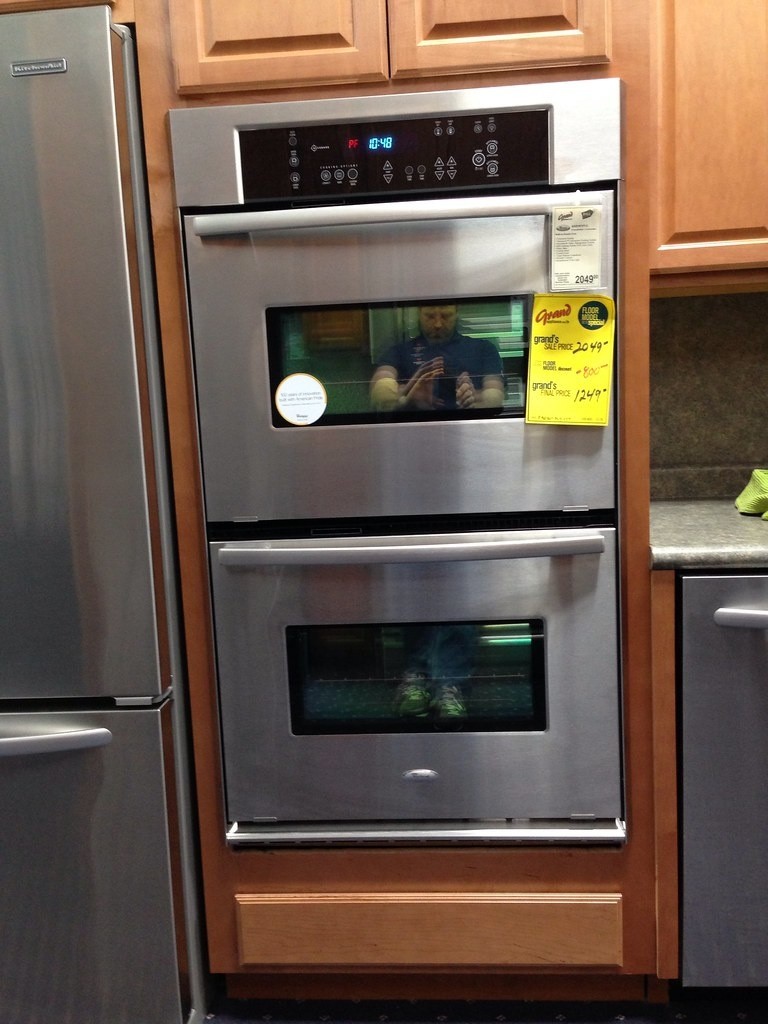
xmin=368 ymin=303 xmax=506 ymax=722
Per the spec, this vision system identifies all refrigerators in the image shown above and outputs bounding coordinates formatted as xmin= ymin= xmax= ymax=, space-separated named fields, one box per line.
xmin=0 ymin=0 xmax=194 ymax=1024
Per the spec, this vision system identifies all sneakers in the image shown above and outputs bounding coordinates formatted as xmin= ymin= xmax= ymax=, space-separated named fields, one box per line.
xmin=392 ymin=673 xmax=431 ymax=718
xmin=434 ymin=682 xmax=467 ymax=720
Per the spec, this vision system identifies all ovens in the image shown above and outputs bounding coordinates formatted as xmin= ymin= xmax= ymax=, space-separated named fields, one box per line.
xmin=167 ymin=78 xmax=632 ymax=848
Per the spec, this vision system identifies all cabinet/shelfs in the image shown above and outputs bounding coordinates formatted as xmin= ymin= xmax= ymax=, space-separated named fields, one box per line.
xmin=165 ymin=0 xmax=613 ymax=96
xmin=649 ymin=0 xmax=768 ymax=273
